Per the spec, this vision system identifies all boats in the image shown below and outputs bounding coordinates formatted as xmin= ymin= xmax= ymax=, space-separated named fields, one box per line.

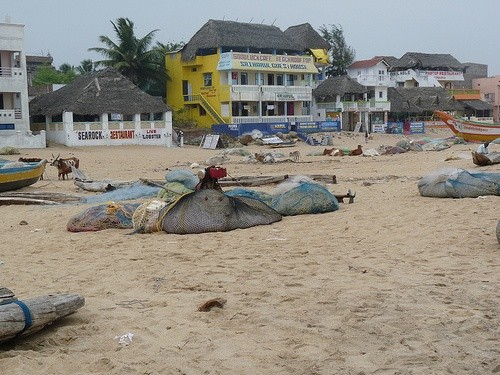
xmin=431 ymin=110 xmax=500 ymax=143
xmin=0 ymin=155 xmax=47 ymax=193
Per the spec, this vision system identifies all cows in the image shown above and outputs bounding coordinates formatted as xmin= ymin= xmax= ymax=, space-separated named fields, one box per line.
xmin=50 ymin=153 xmax=80 ymax=180
xmin=323 ymin=148 xmax=343 ymax=157
xmin=348 ymin=145 xmax=363 ymax=156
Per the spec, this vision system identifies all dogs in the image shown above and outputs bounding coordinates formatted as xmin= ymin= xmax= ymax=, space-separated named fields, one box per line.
xmin=289 ymin=151 xmax=301 ymax=161
